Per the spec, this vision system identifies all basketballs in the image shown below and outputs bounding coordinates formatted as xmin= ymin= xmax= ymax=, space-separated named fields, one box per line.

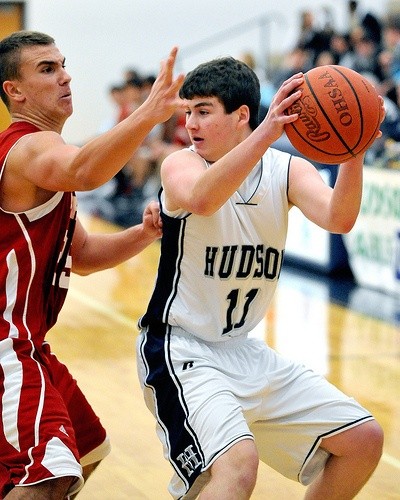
xmin=283 ymin=65 xmax=382 ymax=165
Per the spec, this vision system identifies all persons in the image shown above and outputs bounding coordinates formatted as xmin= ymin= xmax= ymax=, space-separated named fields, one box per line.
xmin=242 ymin=53 xmax=257 ymax=70
xmin=0 ymin=30 xmax=192 ymax=499
xmin=273 ymin=0 xmax=400 ymax=166
xmin=135 ymin=57 xmax=385 ymax=500
xmin=110 ymin=70 xmax=186 ymax=201
